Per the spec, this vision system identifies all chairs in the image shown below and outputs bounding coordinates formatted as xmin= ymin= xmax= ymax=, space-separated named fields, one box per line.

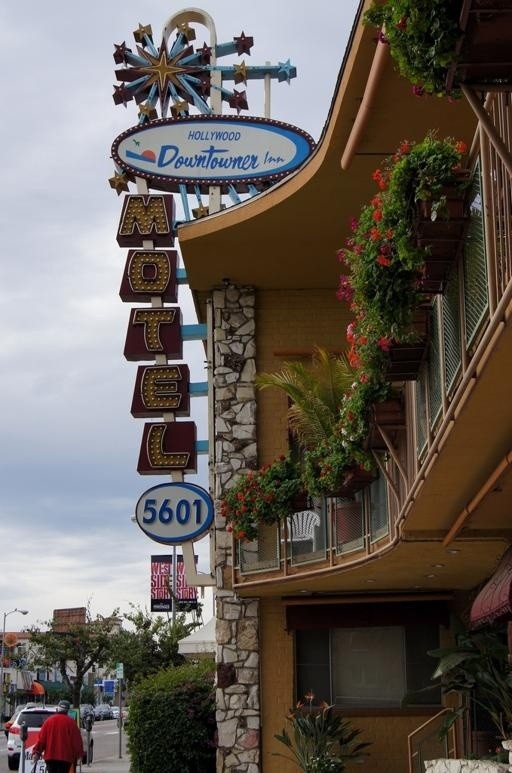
xmin=278 ymin=511 xmax=321 ymax=555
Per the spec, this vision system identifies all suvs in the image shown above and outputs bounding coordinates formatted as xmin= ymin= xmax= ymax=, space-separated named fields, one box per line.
xmin=7 ymin=700 xmax=94 ymax=771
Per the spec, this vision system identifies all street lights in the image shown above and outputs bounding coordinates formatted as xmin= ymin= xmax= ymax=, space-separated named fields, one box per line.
xmin=0 ymin=606 xmax=29 ymax=718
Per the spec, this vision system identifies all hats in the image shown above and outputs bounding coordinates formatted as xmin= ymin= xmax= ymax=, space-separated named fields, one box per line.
xmin=58 ymin=700 xmax=70 ymax=710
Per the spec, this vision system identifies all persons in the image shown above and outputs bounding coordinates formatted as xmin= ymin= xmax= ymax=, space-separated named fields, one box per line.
xmin=31 ymin=701 xmax=84 ymax=773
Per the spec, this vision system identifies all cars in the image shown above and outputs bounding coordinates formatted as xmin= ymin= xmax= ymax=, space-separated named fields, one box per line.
xmin=3 ymin=702 xmax=24 ymax=740
xmin=94 ymin=703 xmax=115 ymax=720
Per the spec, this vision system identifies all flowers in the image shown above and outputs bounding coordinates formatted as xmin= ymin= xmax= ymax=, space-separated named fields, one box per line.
xmin=358 ymin=0 xmax=463 ymax=105
xmin=337 ymin=130 xmax=472 ymax=385
xmin=216 ymin=453 xmax=304 ymax=547
xmin=309 ymin=378 xmax=392 ymax=494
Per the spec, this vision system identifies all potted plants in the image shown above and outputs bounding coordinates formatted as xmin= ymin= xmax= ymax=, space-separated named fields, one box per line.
xmin=422 ymin=625 xmax=512 ymax=764
xmin=256 ymin=346 xmax=376 ymax=547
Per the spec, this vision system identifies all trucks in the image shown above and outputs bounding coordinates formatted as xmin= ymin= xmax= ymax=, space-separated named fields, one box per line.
xmin=111 ymin=705 xmax=120 ymax=718
